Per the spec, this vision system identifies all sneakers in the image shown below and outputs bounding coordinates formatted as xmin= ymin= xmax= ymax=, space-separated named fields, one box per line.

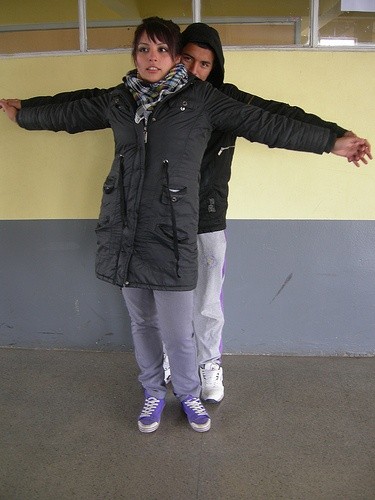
xmin=164 ymin=368 xmax=172 ymax=384
xmin=180 ymin=393 xmax=211 ymax=432
xmin=200 ymin=363 xmax=224 ymax=404
xmin=138 ymin=389 xmax=165 ymax=433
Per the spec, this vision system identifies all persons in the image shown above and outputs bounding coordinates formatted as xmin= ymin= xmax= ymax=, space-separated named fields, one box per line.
xmin=0 ymin=17 xmax=373 ymax=432
xmin=0 ymin=22 xmax=371 ymax=404
